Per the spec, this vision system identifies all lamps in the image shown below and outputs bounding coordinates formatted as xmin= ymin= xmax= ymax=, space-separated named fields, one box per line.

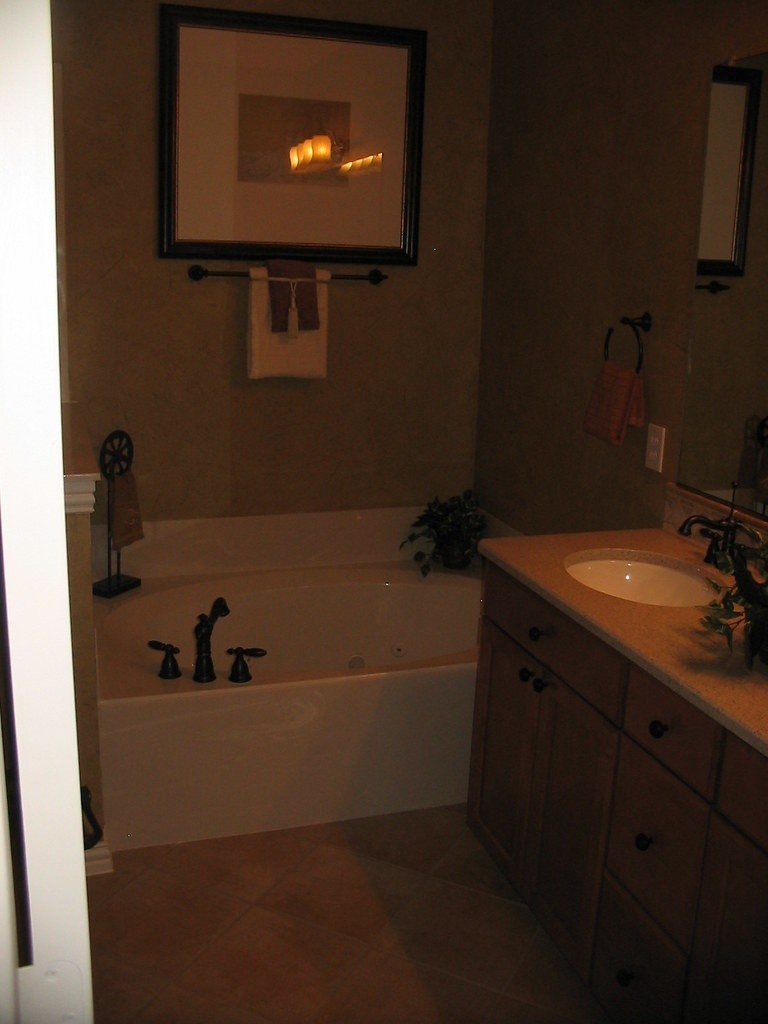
xmin=286 ymin=115 xmax=343 ymax=178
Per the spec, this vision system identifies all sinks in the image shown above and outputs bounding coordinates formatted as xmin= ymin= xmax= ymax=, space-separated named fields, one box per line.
xmin=562 ymin=548 xmax=728 ymax=607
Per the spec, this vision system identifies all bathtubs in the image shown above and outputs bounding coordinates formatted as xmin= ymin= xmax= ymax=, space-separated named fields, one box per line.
xmin=703 ymin=486 xmax=761 ymax=516
xmin=92 ymin=553 xmax=478 ymax=853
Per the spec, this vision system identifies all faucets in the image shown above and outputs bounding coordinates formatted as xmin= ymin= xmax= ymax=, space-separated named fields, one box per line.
xmin=192 ymin=597 xmax=230 ymax=684
xmin=676 ymin=517 xmax=764 ymax=576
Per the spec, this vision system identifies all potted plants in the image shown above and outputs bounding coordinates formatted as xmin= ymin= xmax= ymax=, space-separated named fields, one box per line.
xmin=399 ymin=488 xmax=492 ymax=579
xmin=700 ymin=532 xmax=767 ymax=674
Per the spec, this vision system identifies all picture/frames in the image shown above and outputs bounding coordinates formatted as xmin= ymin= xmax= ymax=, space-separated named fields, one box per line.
xmin=696 ymin=63 xmax=762 ymax=278
xmin=154 ymin=0 xmax=430 ymax=267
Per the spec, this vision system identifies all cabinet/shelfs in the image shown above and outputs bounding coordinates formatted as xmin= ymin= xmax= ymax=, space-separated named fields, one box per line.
xmin=464 ymin=557 xmax=767 ymax=1024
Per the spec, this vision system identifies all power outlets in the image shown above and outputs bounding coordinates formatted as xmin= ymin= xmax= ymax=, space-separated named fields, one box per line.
xmin=645 ymin=421 xmax=667 ymax=475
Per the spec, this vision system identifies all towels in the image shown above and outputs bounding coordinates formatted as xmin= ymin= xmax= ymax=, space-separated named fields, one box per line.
xmin=752 ymin=446 xmax=768 ymax=504
xmin=248 ymin=259 xmax=330 ymax=379
xmin=583 ymin=361 xmax=647 ymax=447
xmin=109 ymin=471 xmax=145 ymax=552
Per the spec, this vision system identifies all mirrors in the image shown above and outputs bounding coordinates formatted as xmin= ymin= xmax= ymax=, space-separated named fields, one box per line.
xmin=675 ymin=48 xmax=768 ymax=520
